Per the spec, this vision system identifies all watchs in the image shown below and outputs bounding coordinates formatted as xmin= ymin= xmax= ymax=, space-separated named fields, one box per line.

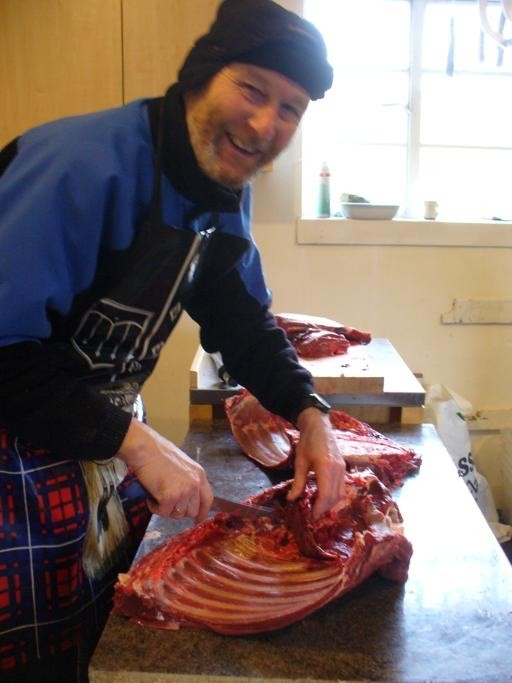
xmin=289 ymin=391 xmax=331 ymax=424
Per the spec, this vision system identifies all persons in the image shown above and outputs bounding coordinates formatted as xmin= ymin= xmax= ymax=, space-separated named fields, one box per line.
xmin=0 ymin=0 xmax=348 ymax=681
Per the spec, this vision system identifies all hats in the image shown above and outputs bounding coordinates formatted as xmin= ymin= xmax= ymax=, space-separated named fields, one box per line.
xmin=209 ymin=0 xmax=333 ymax=102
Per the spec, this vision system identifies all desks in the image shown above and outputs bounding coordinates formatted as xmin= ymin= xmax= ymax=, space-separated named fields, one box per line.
xmin=90 ymin=417 xmax=512 ymax=683
xmin=189 ymin=336 xmax=426 ymax=424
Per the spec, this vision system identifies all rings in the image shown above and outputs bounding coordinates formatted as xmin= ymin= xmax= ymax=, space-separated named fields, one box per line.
xmin=172 ymin=506 xmax=185 ymax=518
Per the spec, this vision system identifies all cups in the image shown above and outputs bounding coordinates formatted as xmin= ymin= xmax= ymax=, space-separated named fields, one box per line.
xmin=422 ymin=200 xmax=440 ymax=220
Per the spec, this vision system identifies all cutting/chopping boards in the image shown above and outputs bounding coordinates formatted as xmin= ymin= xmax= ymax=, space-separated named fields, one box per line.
xmin=298 ymin=343 xmax=384 ymax=396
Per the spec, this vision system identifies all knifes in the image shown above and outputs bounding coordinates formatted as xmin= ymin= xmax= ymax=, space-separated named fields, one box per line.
xmin=122 ymin=479 xmax=279 ymax=525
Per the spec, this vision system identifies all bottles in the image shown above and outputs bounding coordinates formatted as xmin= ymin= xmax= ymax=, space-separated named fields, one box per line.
xmin=316 ymin=160 xmax=331 ymax=218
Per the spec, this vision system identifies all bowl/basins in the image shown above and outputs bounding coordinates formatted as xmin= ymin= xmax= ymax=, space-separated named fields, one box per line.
xmin=341 ymin=202 xmax=401 ymax=220
